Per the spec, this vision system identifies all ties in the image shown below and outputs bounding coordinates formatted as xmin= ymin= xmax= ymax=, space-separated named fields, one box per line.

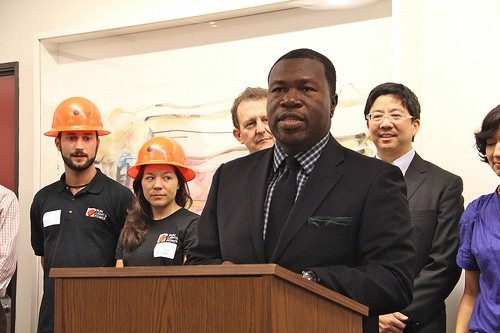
xmin=264 ymin=155 xmax=300 ymax=264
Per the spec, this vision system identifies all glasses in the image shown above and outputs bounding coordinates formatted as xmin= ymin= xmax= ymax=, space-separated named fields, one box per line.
xmin=366 ymin=113 xmax=415 ymax=123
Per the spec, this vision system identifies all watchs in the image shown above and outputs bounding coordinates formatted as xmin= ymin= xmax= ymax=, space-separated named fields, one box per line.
xmin=301 ymin=269 xmax=316 ymax=282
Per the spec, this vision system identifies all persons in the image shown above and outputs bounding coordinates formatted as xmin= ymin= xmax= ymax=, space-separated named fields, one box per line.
xmin=230 ymin=87 xmax=276 ymax=156
xmin=28 ymin=97 xmax=134 ymax=333
xmin=363 ymin=82 xmax=464 ymax=333
xmin=454 ymin=104 xmax=500 ymax=333
xmin=0 ymin=184 xmax=21 ymax=333
xmin=198 ymin=48 xmax=418 ymax=333
xmin=114 ymin=135 xmax=201 ymax=268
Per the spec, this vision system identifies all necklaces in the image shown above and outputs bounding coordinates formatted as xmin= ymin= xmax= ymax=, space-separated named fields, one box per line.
xmin=66 ymin=183 xmax=88 ymax=188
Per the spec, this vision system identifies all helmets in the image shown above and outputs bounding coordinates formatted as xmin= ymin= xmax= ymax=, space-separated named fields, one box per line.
xmin=44 ymin=97 xmax=111 ymax=138
xmin=127 ymin=137 xmax=196 ymax=182
